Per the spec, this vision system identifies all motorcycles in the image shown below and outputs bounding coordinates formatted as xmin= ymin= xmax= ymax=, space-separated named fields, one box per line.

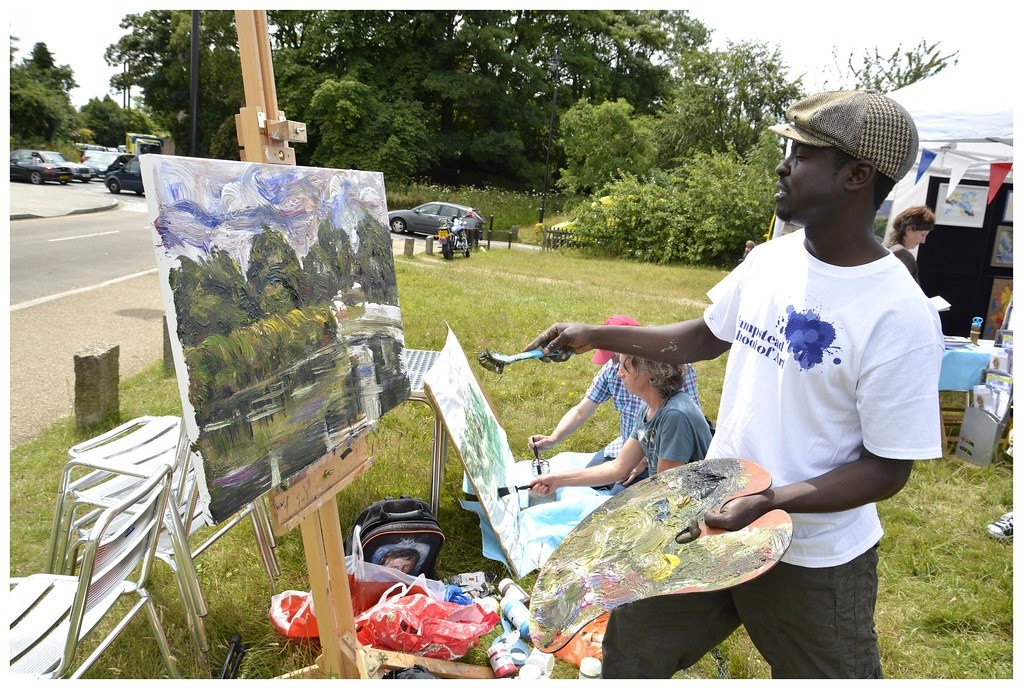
xmin=436 ymin=214 xmax=472 ymax=260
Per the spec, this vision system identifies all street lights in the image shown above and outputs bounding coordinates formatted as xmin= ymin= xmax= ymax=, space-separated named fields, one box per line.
xmin=539 ymin=47 xmax=561 ymax=222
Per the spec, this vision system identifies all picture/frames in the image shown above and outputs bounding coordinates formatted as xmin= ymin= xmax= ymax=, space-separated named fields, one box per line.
xmin=990 ymin=225 xmax=1013 ymax=268
xmin=934 ymin=183 xmax=989 ymax=228
xmin=982 ymin=275 xmax=1013 ymax=339
xmin=1002 ymin=189 xmax=1012 ymax=223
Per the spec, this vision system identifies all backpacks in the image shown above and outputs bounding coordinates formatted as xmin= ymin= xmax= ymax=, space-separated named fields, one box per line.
xmin=343 ymin=496 xmax=445 ymax=580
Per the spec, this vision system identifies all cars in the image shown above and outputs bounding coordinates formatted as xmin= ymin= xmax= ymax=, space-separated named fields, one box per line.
xmin=10 ymin=143 xmax=147 ymax=196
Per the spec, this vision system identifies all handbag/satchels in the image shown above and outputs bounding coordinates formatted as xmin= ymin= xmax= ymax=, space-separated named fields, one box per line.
xmin=269 ymin=525 xmax=446 ymax=637
xmin=354 ymin=575 xmax=501 ymax=662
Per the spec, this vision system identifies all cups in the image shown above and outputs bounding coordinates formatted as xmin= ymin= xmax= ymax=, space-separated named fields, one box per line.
xmin=531 ymin=458 xmax=550 ymax=479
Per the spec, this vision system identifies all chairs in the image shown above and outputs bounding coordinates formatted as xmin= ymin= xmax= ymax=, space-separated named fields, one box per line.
xmin=10 ymin=415 xmax=280 ymax=678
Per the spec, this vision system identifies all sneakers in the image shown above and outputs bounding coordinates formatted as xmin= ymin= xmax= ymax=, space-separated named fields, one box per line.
xmin=987 ymin=512 xmax=1013 ymax=540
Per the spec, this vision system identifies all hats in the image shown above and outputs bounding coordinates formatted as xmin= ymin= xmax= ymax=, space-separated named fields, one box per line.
xmin=591 ymin=315 xmax=640 ymax=365
xmin=768 ymin=90 xmax=919 ymax=182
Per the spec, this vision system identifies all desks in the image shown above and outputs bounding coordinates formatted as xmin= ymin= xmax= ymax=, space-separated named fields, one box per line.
xmin=394 ymin=348 xmax=446 ymax=524
xmin=937 ymin=337 xmax=1013 ymax=455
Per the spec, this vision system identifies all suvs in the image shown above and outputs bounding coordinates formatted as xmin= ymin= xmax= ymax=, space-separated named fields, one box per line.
xmin=387 ymin=201 xmax=487 ymax=239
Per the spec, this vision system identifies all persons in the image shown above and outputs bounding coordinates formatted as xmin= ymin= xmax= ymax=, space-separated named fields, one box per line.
xmin=523 ymin=91 xmax=945 ymax=679
xmin=987 ymin=511 xmax=1013 ymax=538
xmin=881 ymin=205 xmax=935 ymax=287
xmin=530 ymin=351 xmax=713 ymax=496
xmin=528 ymin=316 xmax=700 ymax=500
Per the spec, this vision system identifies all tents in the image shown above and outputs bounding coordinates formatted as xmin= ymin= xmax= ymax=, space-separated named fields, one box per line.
xmin=771 ymin=64 xmax=1014 ymax=241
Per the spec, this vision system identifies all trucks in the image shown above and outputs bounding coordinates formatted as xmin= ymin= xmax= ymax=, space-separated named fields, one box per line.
xmin=126 ymin=132 xmax=164 ymax=154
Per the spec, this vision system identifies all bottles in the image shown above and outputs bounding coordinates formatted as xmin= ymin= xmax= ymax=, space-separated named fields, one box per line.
xmin=489 ymin=578 xmax=531 ymax=678
xmin=578 ymin=657 xmax=602 ymax=679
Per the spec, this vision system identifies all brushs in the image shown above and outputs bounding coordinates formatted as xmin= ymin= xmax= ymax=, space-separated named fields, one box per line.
xmin=532 ymin=437 xmax=542 ymax=475
xmin=478 ymin=348 xmax=574 ymax=373
xmin=498 ymin=485 xmax=531 ymax=498
